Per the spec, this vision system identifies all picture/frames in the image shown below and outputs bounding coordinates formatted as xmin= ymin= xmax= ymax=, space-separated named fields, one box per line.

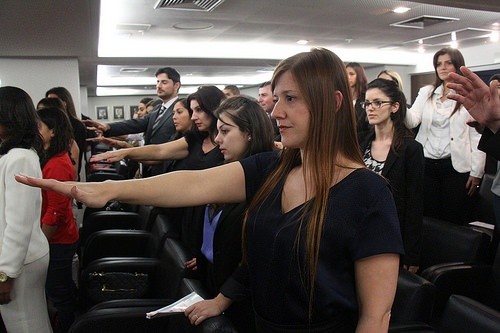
xmin=113 ymin=107 xmax=124 ymax=119
xmin=97 ymin=106 xmax=108 ymax=119
xmin=129 ymin=105 xmax=139 ymax=118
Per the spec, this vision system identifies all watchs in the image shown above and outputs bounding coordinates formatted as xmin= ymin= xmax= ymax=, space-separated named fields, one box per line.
xmin=0 ymin=272 xmax=8 ymax=283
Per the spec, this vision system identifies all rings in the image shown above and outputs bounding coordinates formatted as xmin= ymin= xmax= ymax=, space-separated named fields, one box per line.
xmin=476 ymin=184 xmax=480 ymax=189
xmin=194 ymin=314 xmax=198 ymax=318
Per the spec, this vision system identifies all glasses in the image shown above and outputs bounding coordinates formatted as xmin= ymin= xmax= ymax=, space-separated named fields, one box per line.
xmin=360 ymin=101 xmax=396 ymax=109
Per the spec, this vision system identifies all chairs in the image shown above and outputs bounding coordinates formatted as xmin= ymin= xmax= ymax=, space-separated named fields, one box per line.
xmin=387 ymin=216 xmax=500 ymax=333
xmin=68 ymin=135 xmax=226 ymax=333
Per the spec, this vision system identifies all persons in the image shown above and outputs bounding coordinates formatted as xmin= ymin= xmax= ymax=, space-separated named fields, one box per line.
xmin=38 ymin=107 xmax=79 ymax=333
xmin=404 ymin=47 xmax=487 ymax=222
xmin=37 ymin=60 xmax=500 ymax=195
xmin=90 ymin=86 xmax=226 ymax=259
xmin=14 ymin=49 xmax=402 ymax=333
xmin=80 ymin=67 xmax=183 ymax=142
xmin=359 ymin=77 xmax=426 ymax=276
xmin=185 ymin=95 xmax=277 ymax=326
xmin=0 ymin=86 xmax=51 ymax=333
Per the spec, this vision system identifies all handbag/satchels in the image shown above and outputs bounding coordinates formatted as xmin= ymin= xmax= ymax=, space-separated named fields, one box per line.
xmin=106 ymin=201 xmax=128 ymax=211
xmin=85 ymin=272 xmax=148 ymax=305
